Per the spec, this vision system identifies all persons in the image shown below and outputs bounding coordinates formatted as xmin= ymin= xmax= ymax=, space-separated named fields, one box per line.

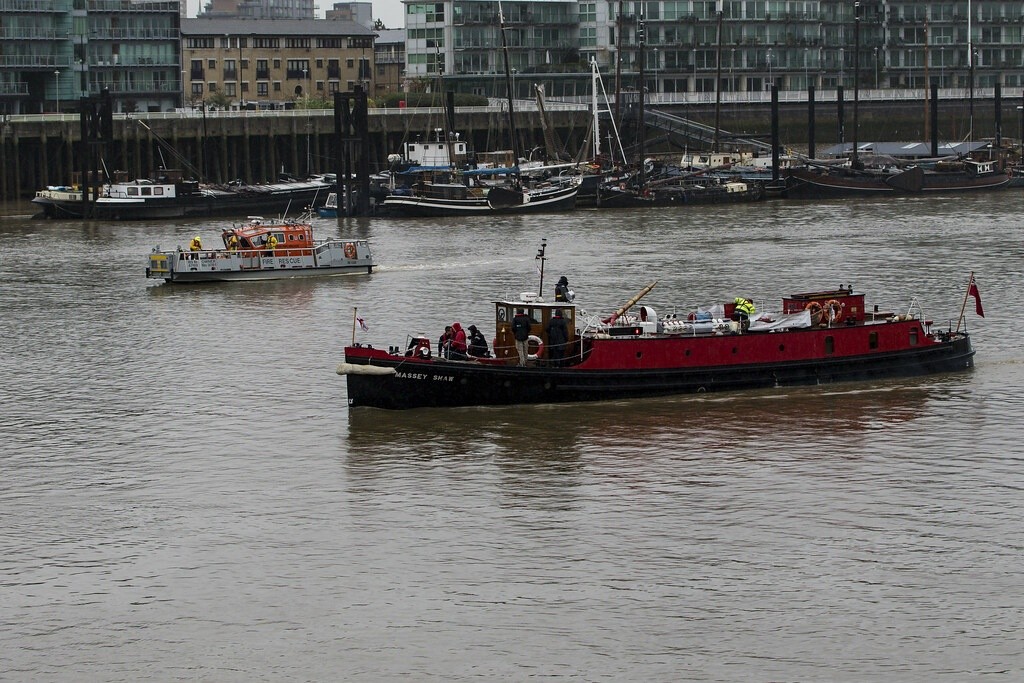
xmin=555 ymin=276 xmax=574 ymax=319
xmin=545 ymin=309 xmax=569 ymax=369
xmin=177 ymin=236 xmax=202 ymax=261
xmin=263 ymin=232 xmax=278 ymax=257
xmin=734 ymin=296 xmax=756 ymax=322
xmin=512 ymin=307 xmax=532 ymax=368
xmin=227 ymin=232 xmax=238 ymax=254
xmin=437 ymin=323 xmax=491 ymax=361
xmin=839 ymin=284 xmax=853 ymax=294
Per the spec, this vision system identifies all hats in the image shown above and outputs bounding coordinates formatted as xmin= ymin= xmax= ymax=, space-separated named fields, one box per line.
xmin=195 ymin=237 xmax=200 ymax=242
xmin=555 ymin=310 xmax=562 ymax=316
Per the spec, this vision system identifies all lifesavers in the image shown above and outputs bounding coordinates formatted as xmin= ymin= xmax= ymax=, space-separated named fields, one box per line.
xmin=344 ymin=243 xmax=356 ymax=258
xmin=824 ymin=300 xmax=843 ymax=323
xmin=523 ymin=335 xmax=545 ymax=360
xmin=804 ymin=301 xmax=824 ymax=326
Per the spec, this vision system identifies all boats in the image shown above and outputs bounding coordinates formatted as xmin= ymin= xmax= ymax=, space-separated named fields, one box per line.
xmin=336 ymin=238 xmax=977 ymax=410
xmin=29 ymin=0 xmax=1024 ymax=220
xmin=145 ymin=184 xmax=379 ymax=284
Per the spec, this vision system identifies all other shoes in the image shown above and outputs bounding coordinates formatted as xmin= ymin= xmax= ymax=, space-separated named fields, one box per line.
xmin=552 ymin=365 xmax=565 ymax=368
xmin=515 ymin=363 xmax=527 ymax=367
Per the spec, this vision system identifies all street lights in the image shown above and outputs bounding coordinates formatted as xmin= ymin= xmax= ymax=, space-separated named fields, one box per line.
xmin=347 ymin=37 xmax=376 ymax=92
xmin=54 ymin=71 xmax=59 ymax=115
xmin=302 ymin=69 xmax=308 ymax=109
xmin=940 ymin=47 xmax=945 ymax=97
xmin=768 ymin=48 xmax=773 ymax=100
xmin=225 ymin=32 xmax=256 ymax=111
xmin=653 ymin=48 xmax=658 ymax=102
xmin=873 ymin=47 xmax=879 ymax=99
xmin=66 ymin=31 xmax=97 ymax=97
xmin=804 ymin=48 xmax=809 ymax=100
xmin=908 ymin=49 xmax=913 ymax=98
xmin=510 ymin=66 xmax=517 ymax=105
xmin=730 ymin=47 xmax=737 ymax=102
xmin=839 ymin=48 xmax=843 ymax=85
xmin=692 ymin=48 xmax=698 ymax=101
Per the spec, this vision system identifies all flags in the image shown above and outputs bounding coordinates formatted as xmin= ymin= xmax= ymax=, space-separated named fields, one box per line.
xmin=969 ymin=276 xmax=985 ymax=318
